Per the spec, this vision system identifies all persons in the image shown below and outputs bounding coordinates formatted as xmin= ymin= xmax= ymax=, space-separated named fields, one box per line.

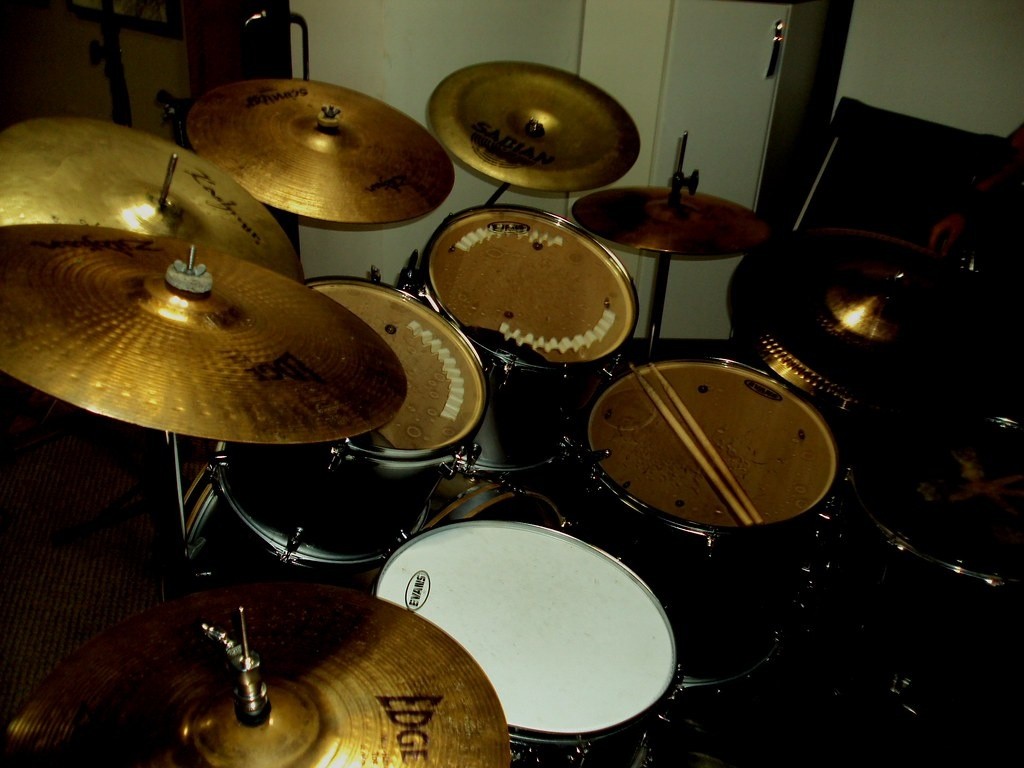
xmin=928 ymin=120 xmax=1024 ymax=256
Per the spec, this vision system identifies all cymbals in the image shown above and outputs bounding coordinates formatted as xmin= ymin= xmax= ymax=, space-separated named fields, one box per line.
xmin=0 ymin=583 xmax=509 ymax=768
xmin=729 ymin=226 xmax=1024 ymax=415
xmin=573 ymin=187 xmax=768 ymax=253
xmin=428 ymin=61 xmax=642 ymax=193
xmin=187 ymin=79 xmax=457 ymax=220
xmin=0 ymin=116 xmax=309 ymax=286
xmin=1 ymin=223 xmax=409 ymax=446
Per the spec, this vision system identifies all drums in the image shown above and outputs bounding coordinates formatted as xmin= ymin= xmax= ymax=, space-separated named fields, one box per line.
xmin=375 ymin=520 xmax=687 ymax=768
xmin=407 ymin=204 xmax=643 ymax=477
xmin=792 ymin=419 xmax=1024 ymax=724
xmin=210 ymin=276 xmax=489 ymax=568
xmin=584 ymin=360 xmax=841 ymax=676
